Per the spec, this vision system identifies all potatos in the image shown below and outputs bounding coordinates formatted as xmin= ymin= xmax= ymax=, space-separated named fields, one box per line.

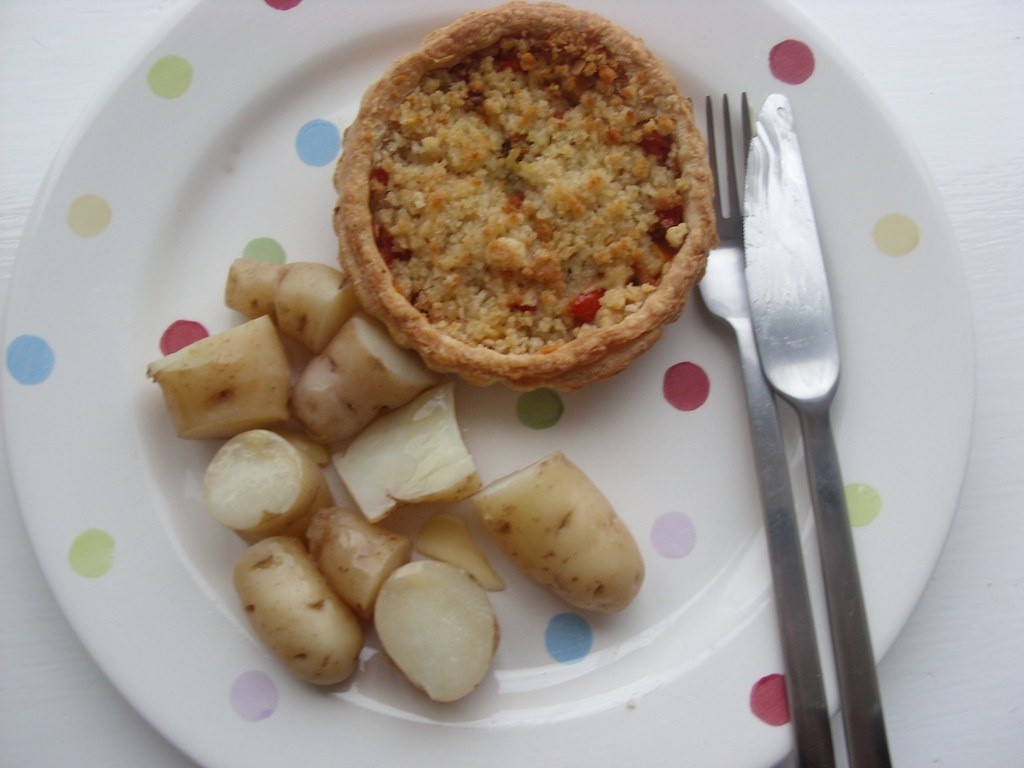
xmin=144 ymin=255 xmax=646 ymax=703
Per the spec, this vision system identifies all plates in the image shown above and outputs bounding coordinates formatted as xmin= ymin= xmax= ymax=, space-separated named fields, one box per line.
xmin=0 ymin=0 xmax=974 ymax=768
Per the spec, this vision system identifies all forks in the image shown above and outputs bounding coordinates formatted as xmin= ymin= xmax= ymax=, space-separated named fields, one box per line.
xmin=688 ymin=94 xmax=835 ymax=768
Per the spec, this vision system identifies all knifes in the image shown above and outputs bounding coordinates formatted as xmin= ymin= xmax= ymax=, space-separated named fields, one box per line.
xmin=742 ymin=93 xmax=892 ymax=768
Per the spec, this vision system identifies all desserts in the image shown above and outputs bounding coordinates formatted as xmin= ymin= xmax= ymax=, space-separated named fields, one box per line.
xmin=332 ymin=0 xmax=720 ymax=392
